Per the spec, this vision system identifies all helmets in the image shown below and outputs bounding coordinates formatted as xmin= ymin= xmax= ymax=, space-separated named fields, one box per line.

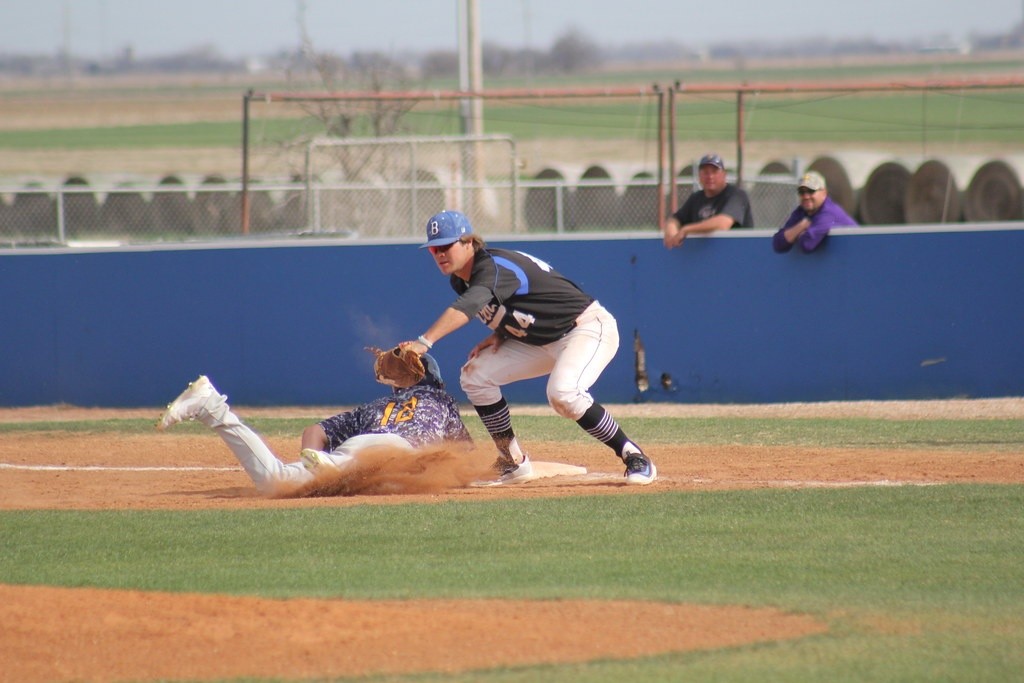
xmin=390 ymin=353 xmax=444 ymax=395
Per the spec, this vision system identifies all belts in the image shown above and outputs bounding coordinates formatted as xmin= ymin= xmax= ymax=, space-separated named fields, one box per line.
xmin=556 ymin=321 xmax=578 ymax=340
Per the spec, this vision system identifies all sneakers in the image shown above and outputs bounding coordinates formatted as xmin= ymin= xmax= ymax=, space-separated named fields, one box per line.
xmin=465 ymin=454 xmax=533 ymax=488
xmin=623 ymin=451 xmax=657 ymax=485
xmin=299 ymin=448 xmax=345 ymax=493
xmin=152 ymin=375 xmax=215 ymax=431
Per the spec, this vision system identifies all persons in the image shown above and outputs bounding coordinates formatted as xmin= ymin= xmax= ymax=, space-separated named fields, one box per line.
xmin=663 ymin=154 xmax=753 ymax=250
xmin=154 ymin=353 xmax=477 ymax=499
xmin=373 ymin=209 xmax=657 ymax=488
xmin=773 ymin=171 xmax=856 ymax=255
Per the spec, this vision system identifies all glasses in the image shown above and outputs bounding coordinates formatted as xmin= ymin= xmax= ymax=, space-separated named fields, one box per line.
xmin=427 ymin=242 xmax=456 ymax=255
xmin=796 ymin=188 xmax=815 ymax=196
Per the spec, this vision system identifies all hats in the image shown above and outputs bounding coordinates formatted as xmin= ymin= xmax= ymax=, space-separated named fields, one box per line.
xmin=697 ymin=152 xmax=725 ymax=170
xmin=417 ymin=209 xmax=472 ymax=250
xmin=796 ymin=170 xmax=826 ymax=190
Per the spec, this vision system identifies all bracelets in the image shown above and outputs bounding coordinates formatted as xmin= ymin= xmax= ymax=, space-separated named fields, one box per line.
xmin=418 ymin=335 xmax=433 ymax=348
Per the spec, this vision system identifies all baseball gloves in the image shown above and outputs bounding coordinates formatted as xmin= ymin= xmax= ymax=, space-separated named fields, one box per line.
xmin=363 ymin=340 xmax=426 ymax=389
